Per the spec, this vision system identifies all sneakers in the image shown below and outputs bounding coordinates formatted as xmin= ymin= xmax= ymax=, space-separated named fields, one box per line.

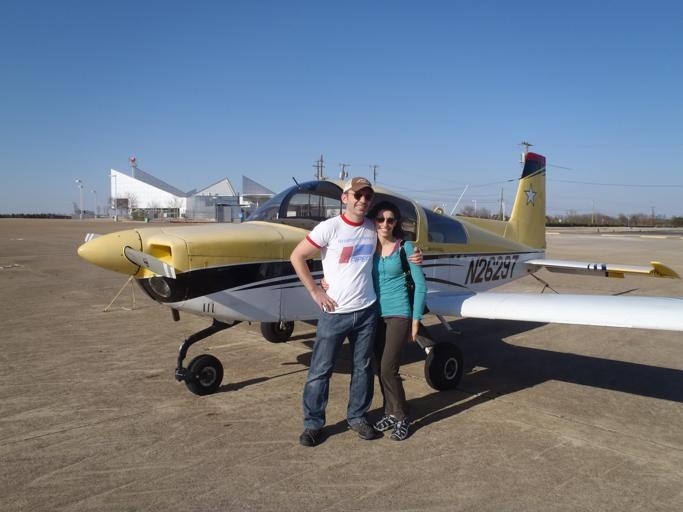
xmin=300 ymin=429 xmax=321 ymax=446
xmin=373 ymin=415 xmax=398 ymax=432
xmin=391 ymin=417 xmax=410 ymax=441
xmin=351 ymin=421 xmax=376 ymax=440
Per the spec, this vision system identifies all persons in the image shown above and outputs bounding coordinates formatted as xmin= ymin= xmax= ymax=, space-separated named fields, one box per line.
xmin=320 ymin=201 xmax=424 ymax=440
xmin=291 ymin=174 xmax=424 ymax=448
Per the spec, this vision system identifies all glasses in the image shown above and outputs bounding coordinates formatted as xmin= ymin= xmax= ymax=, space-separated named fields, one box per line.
xmin=376 ymin=215 xmax=393 ymax=224
xmin=354 ymin=191 xmax=373 ymax=201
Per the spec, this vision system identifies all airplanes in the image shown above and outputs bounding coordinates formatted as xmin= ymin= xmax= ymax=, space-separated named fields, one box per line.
xmin=76 ymin=153 xmax=682 ymax=396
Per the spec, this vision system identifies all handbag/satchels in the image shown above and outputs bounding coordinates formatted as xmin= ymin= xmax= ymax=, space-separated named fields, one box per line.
xmin=400 ymin=240 xmax=430 ymax=314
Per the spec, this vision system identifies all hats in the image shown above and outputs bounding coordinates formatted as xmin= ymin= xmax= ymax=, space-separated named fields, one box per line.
xmin=343 ymin=177 xmax=373 ymax=193
